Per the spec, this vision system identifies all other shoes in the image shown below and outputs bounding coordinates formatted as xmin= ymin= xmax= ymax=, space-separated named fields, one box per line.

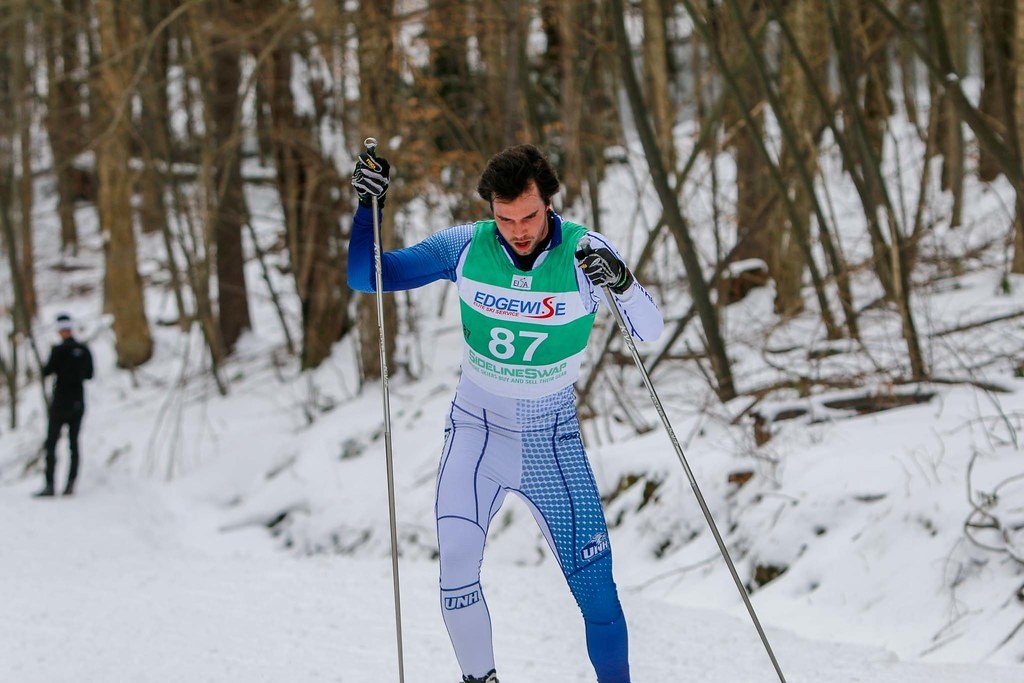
xmin=37 ymin=488 xmax=54 ymax=496
xmin=63 ymin=487 xmax=72 ymax=495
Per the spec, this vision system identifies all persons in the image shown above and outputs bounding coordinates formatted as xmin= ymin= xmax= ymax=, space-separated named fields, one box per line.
xmin=36 ymin=314 xmax=95 ymax=496
xmin=348 ymin=143 xmax=664 ymax=683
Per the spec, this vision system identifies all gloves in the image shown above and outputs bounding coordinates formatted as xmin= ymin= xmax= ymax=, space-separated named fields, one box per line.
xmin=351 ymin=146 xmax=390 ymax=209
xmin=576 ymin=248 xmax=633 ymax=294
